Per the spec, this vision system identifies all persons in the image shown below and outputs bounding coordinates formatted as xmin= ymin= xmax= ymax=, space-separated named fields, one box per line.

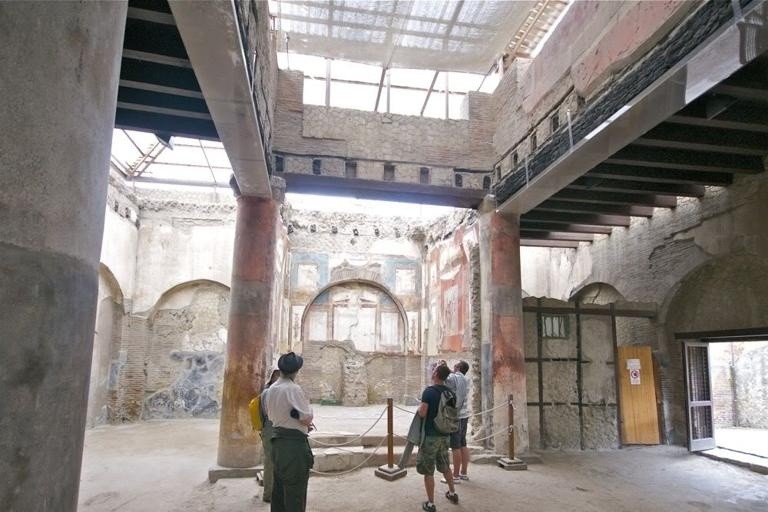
xmin=259 ymin=369 xmax=280 ymax=502
xmin=417 ymin=363 xmax=458 ymax=511
xmin=437 ymin=359 xmax=470 ymax=484
xmin=267 ymin=352 xmax=314 ymax=511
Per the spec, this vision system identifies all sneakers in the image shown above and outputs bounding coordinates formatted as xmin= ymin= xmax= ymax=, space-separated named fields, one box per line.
xmin=446 ymin=490 xmax=458 ymax=502
xmin=459 ymin=474 xmax=468 ymax=480
xmin=424 ymin=501 xmax=436 ymax=512
xmin=441 ymin=477 xmax=460 ymax=483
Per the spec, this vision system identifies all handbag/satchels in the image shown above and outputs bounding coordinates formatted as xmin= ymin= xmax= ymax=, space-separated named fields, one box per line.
xmin=250 ymin=394 xmax=264 ymax=430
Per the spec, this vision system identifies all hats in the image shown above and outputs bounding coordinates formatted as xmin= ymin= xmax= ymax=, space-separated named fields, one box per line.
xmin=265 ymin=366 xmax=279 ymax=384
xmin=278 ymin=352 xmax=303 ymax=373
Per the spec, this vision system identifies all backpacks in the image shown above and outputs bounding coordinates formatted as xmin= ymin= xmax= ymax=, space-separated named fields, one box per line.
xmin=429 ymin=384 xmax=459 ymax=433
